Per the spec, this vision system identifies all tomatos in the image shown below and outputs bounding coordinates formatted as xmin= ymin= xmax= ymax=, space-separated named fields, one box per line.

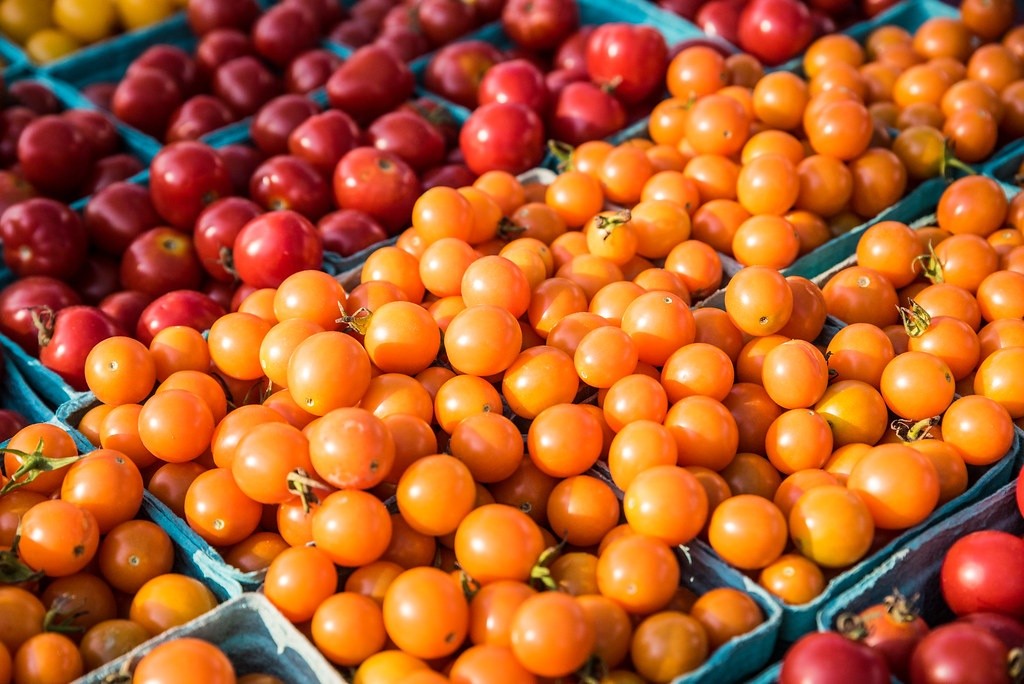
xmin=0 ymin=0 xmax=1024 ymax=684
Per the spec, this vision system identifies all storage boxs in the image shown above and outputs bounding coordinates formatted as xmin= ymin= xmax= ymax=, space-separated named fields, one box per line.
xmin=0 ymin=0 xmax=1024 ymax=684
